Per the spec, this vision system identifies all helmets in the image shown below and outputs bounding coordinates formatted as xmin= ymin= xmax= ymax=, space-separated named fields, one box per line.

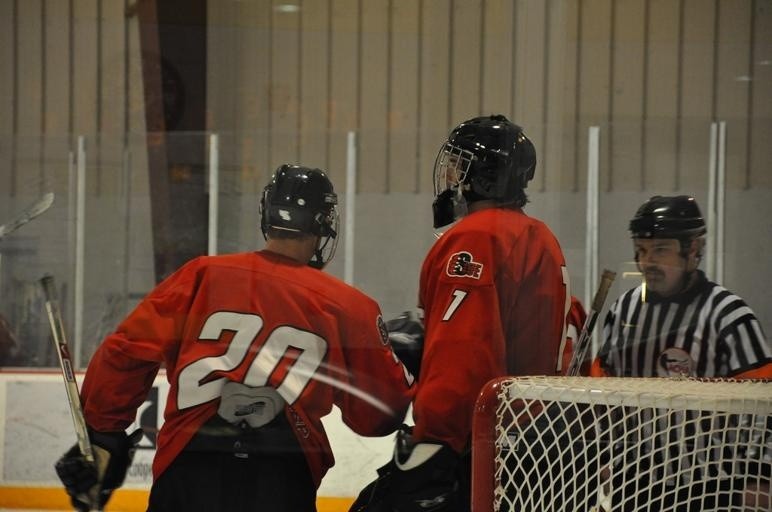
xmin=432 ymin=116 xmax=535 ymax=228
xmin=628 ymin=194 xmax=706 ymax=267
xmin=259 ymin=164 xmax=338 ymax=268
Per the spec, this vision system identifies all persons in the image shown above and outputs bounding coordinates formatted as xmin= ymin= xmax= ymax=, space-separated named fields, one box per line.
xmin=52 ymin=164 xmax=418 ymax=511
xmin=348 ymin=112 xmax=574 ymax=512
xmin=385 ymin=294 xmax=601 ymax=510
xmin=590 ymin=195 xmax=771 ymax=511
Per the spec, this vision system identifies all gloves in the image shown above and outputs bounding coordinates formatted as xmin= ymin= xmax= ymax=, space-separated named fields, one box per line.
xmin=393 ymin=424 xmax=470 ymax=511
xmin=56 ymin=429 xmax=142 ymax=511
xmin=387 ymin=310 xmax=425 ymax=379
